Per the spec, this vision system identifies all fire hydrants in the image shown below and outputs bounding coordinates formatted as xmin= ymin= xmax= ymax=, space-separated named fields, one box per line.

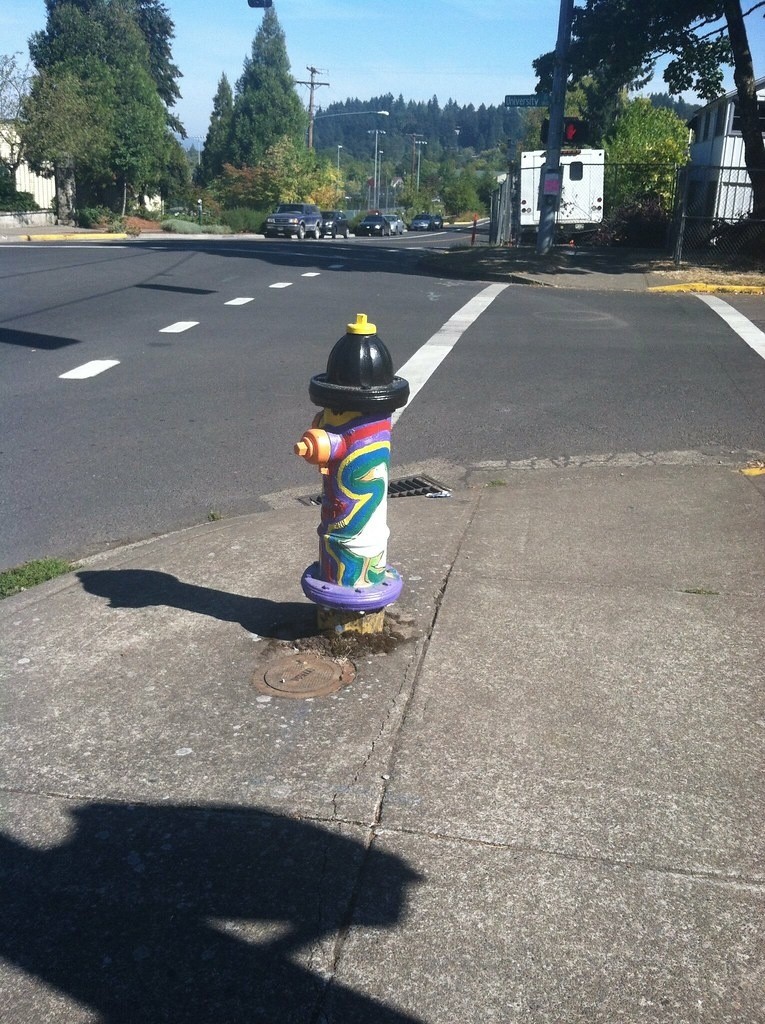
xmin=290 ymin=311 xmax=412 ymax=638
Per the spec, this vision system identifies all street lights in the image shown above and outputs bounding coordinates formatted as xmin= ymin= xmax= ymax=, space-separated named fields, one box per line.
xmin=298 ymin=68 xmax=390 ymax=149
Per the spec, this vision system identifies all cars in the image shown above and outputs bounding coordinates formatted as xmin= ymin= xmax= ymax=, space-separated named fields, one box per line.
xmin=410 ymin=213 xmax=435 ymax=232
xmin=430 ymin=216 xmax=443 ymax=229
xmin=320 ymin=210 xmax=350 ymax=239
xmin=382 ymin=215 xmax=404 ymax=235
xmin=355 ymin=214 xmax=392 ymax=237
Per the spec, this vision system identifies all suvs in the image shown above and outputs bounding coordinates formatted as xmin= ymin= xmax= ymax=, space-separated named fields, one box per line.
xmin=264 ymin=203 xmax=324 ymax=240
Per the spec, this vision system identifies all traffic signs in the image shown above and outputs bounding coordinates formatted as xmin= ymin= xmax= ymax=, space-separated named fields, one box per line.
xmin=504 ymin=93 xmax=550 ymax=108
